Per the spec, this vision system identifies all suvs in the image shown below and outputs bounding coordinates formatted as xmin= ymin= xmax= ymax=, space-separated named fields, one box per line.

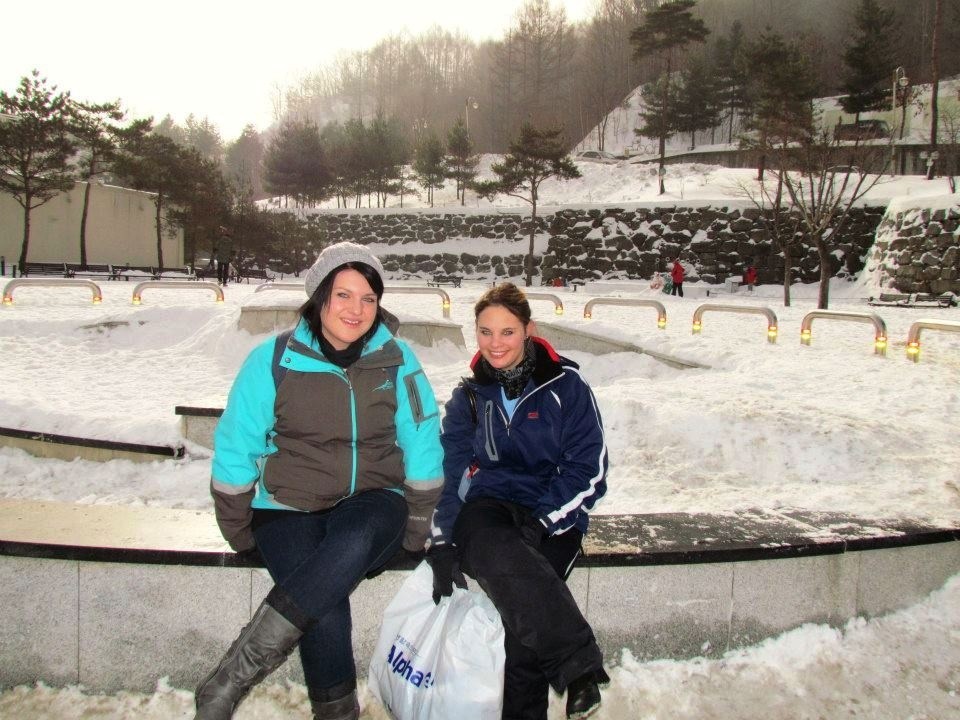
xmin=835 ymin=118 xmax=890 ymax=141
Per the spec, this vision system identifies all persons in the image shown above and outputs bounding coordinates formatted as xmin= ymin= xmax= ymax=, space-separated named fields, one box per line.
xmin=210 ymin=233 xmax=235 ymax=286
xmin=650 ymin=271 xmax=673 ymax=294
xmin=192 ymin=241 xmax=444 ymax=720
xmin=426 ymin=281 xmax=610 ymax=720
xmin=671 ymin=260 xmax=684 ymax=297
xmin=546 ymin=271 xmax=568 ymax=286
xmin=747 ymin=266 xmax=756 ymax=291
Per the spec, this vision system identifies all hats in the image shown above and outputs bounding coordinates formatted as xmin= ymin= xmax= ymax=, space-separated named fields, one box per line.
xmin=304 ymin=243 xmax=384 ymax=299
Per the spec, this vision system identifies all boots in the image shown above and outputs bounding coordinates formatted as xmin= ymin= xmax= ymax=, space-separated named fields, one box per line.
xmin=196 ymin=597 xmax=304 ymax=720
xmin=311 ymin=690 xmax=360 ymax=720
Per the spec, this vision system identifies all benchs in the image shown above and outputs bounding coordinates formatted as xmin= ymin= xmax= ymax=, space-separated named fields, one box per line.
xmin=19 ymin=262 xmax=276 ymax=284
xmin=427 ymin=274 xmax=463 ymax=288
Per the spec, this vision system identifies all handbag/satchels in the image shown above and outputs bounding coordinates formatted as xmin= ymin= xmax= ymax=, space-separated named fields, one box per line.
xmin=367 ymin=559 xmax=507 ymax=720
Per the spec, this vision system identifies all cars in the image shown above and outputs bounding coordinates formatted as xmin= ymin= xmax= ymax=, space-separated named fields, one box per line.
xmin=574 ymin=151 xmax=619 ymax=164
xmin=824 ymin=165 xmax=869 ymax=175
xmin=194 ymin=258 xmax=238 ymax=277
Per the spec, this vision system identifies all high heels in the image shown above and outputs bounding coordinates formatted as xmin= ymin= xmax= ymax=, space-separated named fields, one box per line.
xmin=566 ymin=665 xmax=610 ymax=720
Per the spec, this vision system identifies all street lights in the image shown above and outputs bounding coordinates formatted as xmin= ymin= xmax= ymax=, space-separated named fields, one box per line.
xmin=887 ymin=66 xmax=910 ymax=176
xmin=465 ymin=96 xmax=479 ymax=143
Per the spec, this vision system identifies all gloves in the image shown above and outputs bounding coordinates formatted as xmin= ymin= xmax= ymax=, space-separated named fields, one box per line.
xmin=520 ymin=518 xmax=545 ymax=550
xmin=426 ymin=542 xmax=470 ymax=607
